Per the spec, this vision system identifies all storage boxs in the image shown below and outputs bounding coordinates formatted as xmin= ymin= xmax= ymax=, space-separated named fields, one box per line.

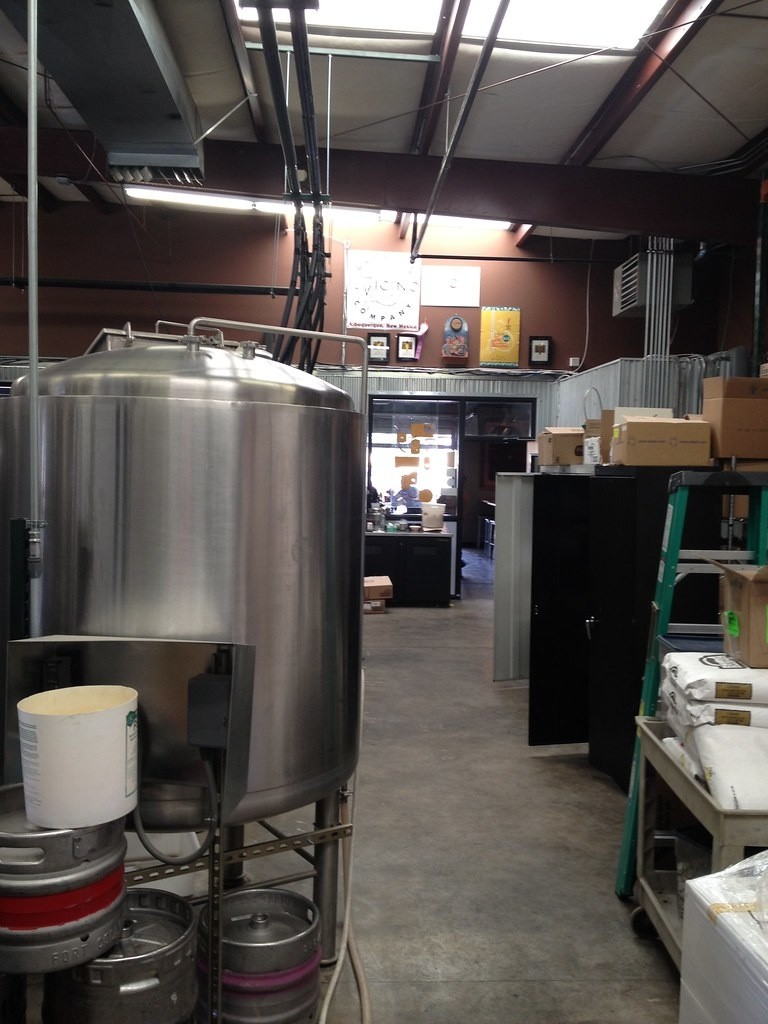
xmin=363 ymin=599 xmax=386 ymax=614
xmin=537 ymin=376 xmax=768 ymax=468
xmin=363 ymin=575 xmax=393 ymax=600
xmin=696 ymin=554 xmax=768 ymax=669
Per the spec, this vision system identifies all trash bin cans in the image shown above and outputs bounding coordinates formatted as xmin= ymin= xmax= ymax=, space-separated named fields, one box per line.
xmin=421 ymin=502 xmax=446 ymax=527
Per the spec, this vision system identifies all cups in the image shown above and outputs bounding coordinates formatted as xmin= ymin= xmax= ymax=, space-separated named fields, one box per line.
xmin=17 ymin=685 xmax=138 ymax=828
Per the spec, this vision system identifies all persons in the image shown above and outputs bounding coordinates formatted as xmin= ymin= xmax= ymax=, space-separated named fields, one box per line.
xmin=395 ymin=486 xmax=422 ymax=507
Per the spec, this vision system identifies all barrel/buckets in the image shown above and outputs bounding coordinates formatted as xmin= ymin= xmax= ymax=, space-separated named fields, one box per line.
xmin=421 ymin=502 xmax=446 ymax=527
xmin=201 ymin=887 xmax=322 ymax=1024
xmin=68 ymin=888 xmax=200 ymax=1024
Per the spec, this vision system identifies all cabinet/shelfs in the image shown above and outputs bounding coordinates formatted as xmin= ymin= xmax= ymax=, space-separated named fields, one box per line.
xmin=634 ymin=715 xmax=768 ymax=968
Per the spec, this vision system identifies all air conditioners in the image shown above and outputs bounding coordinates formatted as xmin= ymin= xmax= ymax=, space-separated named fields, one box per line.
xmin=611 ymin=244 xmax=694 ymax=317
